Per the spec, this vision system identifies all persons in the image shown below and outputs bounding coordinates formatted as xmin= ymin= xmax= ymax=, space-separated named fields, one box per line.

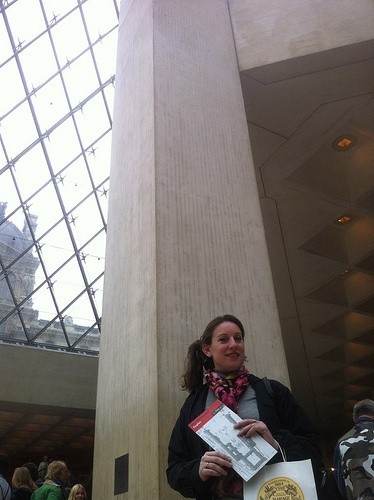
xmin=31 ymin=460 xmax=68 ymax=500
xmin=38 ymin=455 xmax=48 ymax=477
xmin=9 ymin=467 xmax=38 ymax=500
xmin=320 ymin=463 xmax=340 ymax=500
xmin=166 ymin=315 xmax=321 ymax=500
xmin=332 ymin=399 xmax=374 ymax=500
xmin=68 ymin=483 xmax=87 ymax=500
xmin=0 ymin=453 xmax=11 ymax=500
xmin=25 ymin=462 xmax=43 ymax=487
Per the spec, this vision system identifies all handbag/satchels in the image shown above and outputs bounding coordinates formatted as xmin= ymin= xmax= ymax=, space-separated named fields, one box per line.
xmin=243 ymin=440 xmax=318 ymax=500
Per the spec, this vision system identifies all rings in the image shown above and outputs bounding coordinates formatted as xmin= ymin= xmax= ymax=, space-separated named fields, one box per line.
xmin=206 ymin=462 xmax=208 ymax=468
